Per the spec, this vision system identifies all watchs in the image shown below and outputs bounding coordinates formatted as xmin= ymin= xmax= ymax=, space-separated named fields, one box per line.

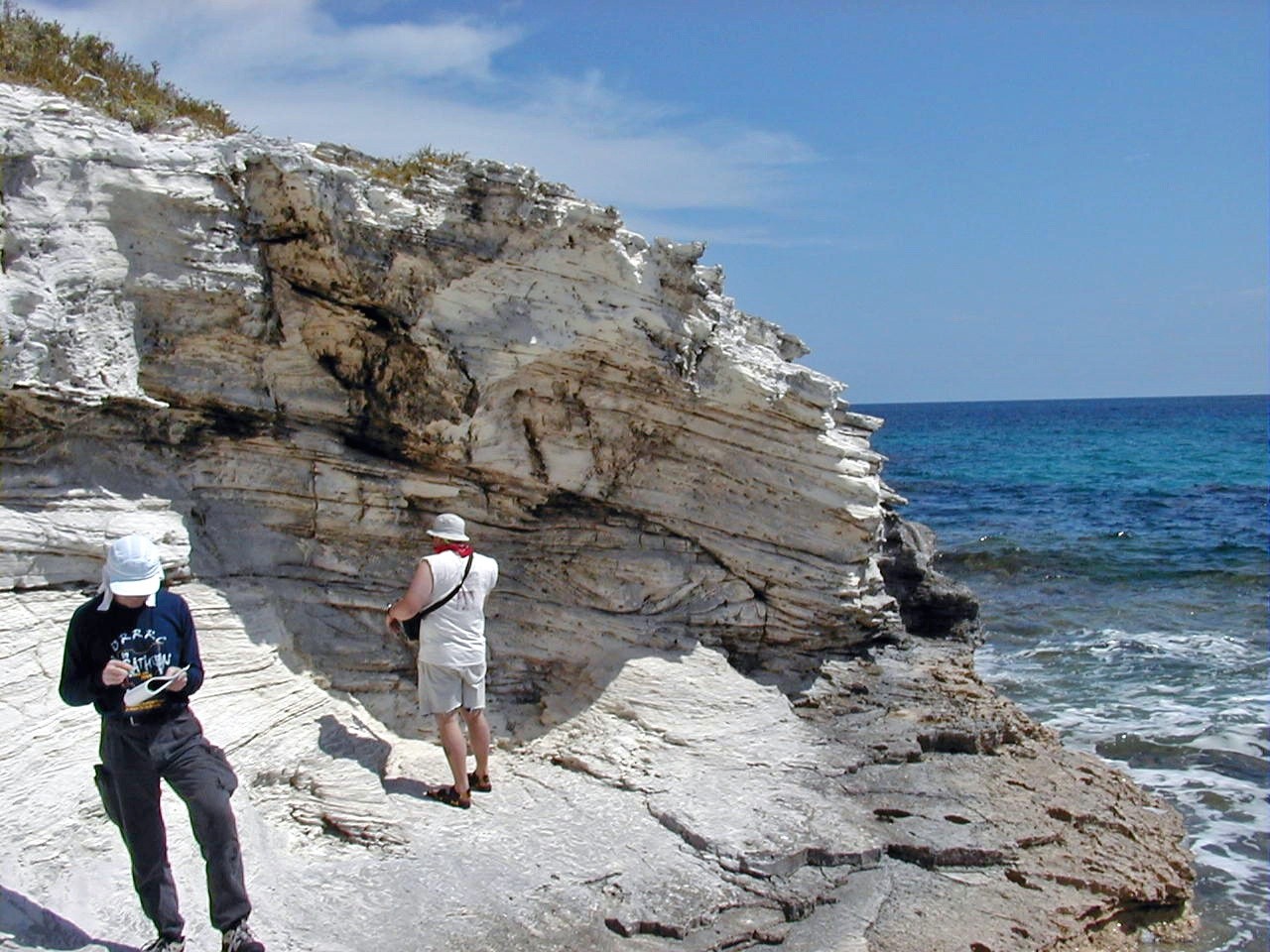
xmin=384 ymin=602 xmax=398 ymax=617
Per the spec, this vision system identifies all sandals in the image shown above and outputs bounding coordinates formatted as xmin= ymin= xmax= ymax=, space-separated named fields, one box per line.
xmin=428 ymin=785 xmax=470 ymax=808
xmin=467 ymin=771 xmax=491 ymax=791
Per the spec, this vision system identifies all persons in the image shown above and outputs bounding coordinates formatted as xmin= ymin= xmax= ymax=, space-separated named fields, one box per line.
xmin=58 ymin=535 xmax=265 ymax=952
xmin=386 ymin=509 xmax=499 ymax=808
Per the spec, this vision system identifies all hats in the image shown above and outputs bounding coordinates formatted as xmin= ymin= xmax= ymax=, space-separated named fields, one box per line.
xmin=97 ymin=535 xmax=165 ymax=611
xmin=426 ymin=513 xmax=469 ymax=541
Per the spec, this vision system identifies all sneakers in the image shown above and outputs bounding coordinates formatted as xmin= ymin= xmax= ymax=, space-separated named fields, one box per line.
xmin=222 ymin=919 xmax=265 ymax=952
xmin=139 ymin=936 xmax=185 ymax=952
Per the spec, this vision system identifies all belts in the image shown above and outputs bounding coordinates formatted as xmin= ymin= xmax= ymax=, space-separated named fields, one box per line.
xmin=109 ymin=710 xmax=172 ymax=726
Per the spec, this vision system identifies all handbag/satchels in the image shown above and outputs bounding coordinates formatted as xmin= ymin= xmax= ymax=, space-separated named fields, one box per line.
xmin=403 ymin=612 xmax=421 ymax=640
